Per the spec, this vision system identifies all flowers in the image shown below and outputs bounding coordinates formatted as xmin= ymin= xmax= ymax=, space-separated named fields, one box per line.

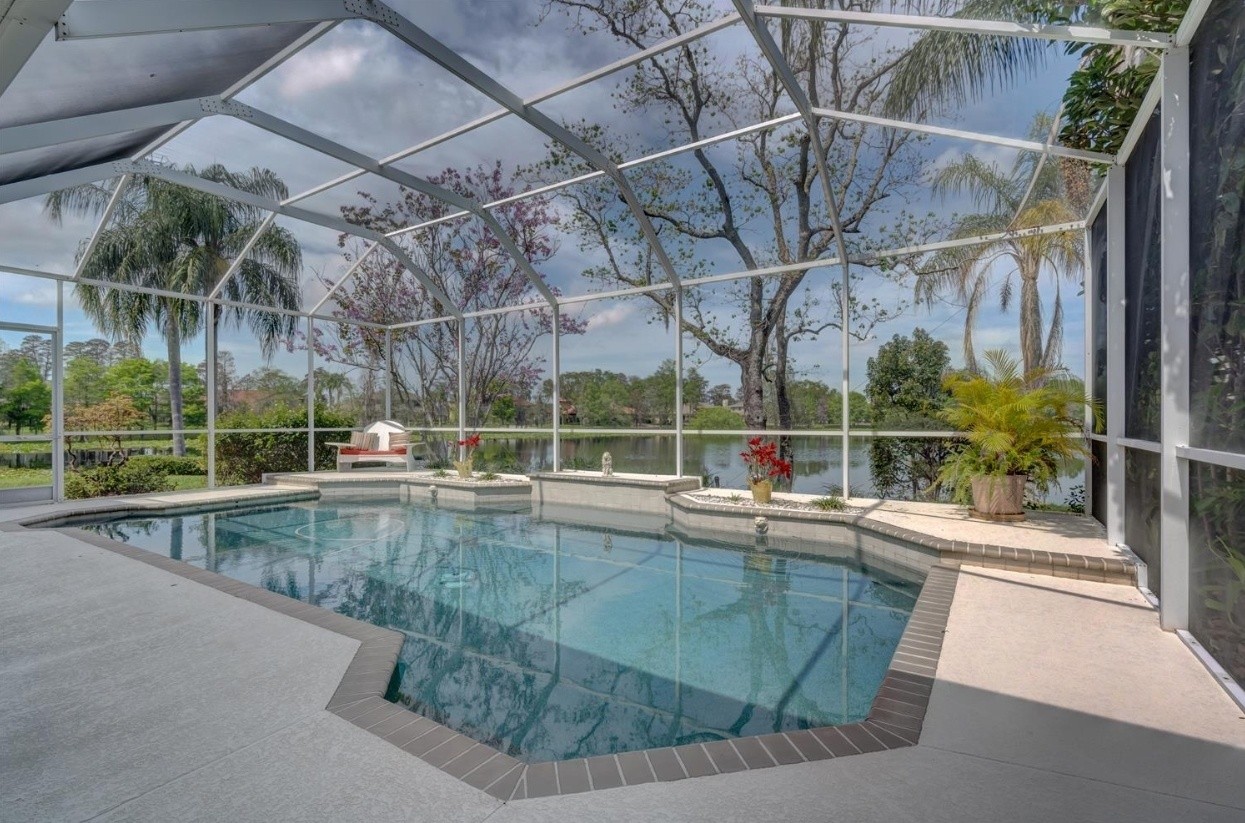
xmin=448 ymin=433 xmax=480 ymax=461
xmin=739 ymin=437 xmax=792 ymax=482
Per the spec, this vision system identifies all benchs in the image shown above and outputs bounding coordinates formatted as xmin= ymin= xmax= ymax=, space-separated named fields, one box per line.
xmin=326 ymin=419 xmax=425 ymax=473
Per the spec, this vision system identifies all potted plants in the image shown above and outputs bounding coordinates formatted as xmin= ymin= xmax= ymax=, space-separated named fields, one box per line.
xmin=919 ymin=349 xmax=1106 ymax=523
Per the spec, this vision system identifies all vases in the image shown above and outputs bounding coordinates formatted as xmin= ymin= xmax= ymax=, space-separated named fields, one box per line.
xmin=454 ymin=459 xmax=473 ymax=479
xmin=748 ymin=479 xmax=773 ymax=504
